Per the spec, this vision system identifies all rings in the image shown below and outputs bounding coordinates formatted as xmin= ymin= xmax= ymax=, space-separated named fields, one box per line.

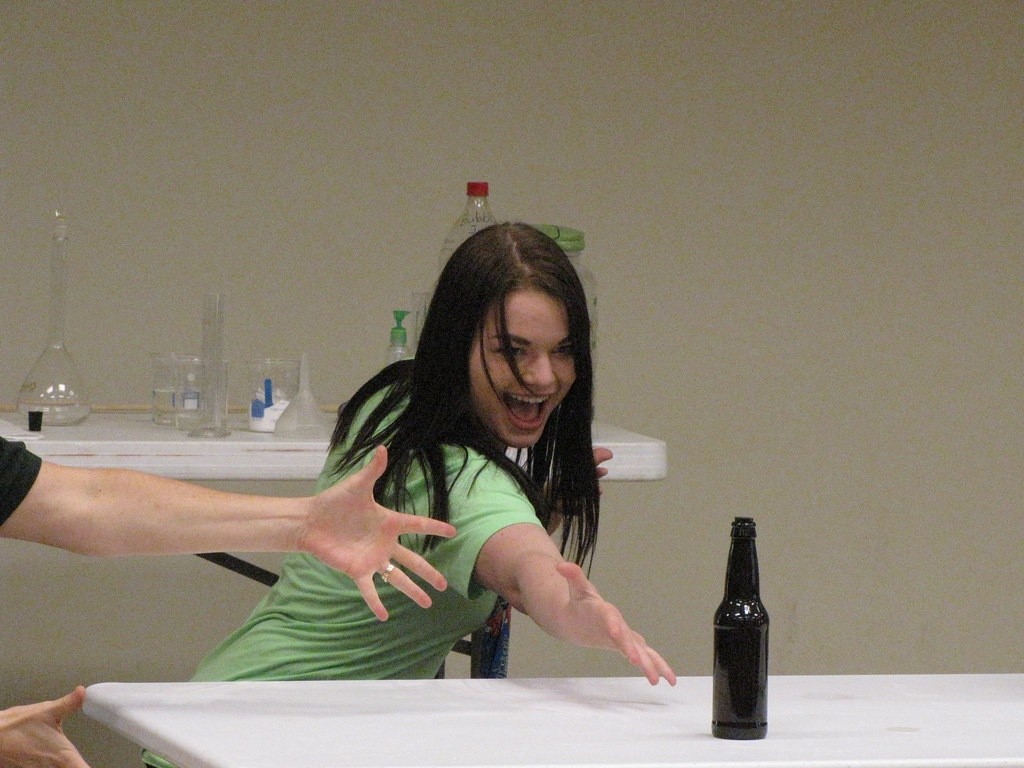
xmin=382 ymin=563 xmax=396 ymax=582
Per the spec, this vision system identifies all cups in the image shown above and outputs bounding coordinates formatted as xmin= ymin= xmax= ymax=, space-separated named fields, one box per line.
xmin=171 ymin=357 xmax=233 ymax=430
xmin=144 ymin=350 xmax=187 ymax=425
xmin=245 ymin=357 xmax=300 ymax=434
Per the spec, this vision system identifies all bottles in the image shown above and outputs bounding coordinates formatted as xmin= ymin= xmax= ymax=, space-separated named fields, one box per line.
xmin=710 ymin=516 xmax=769 ymax=739
xmin=436 ymin=179 xmax=497 ymax=275
xmin=14 ymin=214 xmax=94 ymax=427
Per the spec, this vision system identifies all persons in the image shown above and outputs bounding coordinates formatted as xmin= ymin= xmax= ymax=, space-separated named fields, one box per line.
xmin=140 ymin=221 xmax=676 ymax=768
xmin=0 ymin=436 xmax=456 ymax=768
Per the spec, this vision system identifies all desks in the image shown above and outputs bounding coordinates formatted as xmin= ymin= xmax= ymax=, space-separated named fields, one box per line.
xmin=63 ymin=670 xmax=1024 ymax=768
xmin=0 ymin=414 xmax=667 ymax=680
